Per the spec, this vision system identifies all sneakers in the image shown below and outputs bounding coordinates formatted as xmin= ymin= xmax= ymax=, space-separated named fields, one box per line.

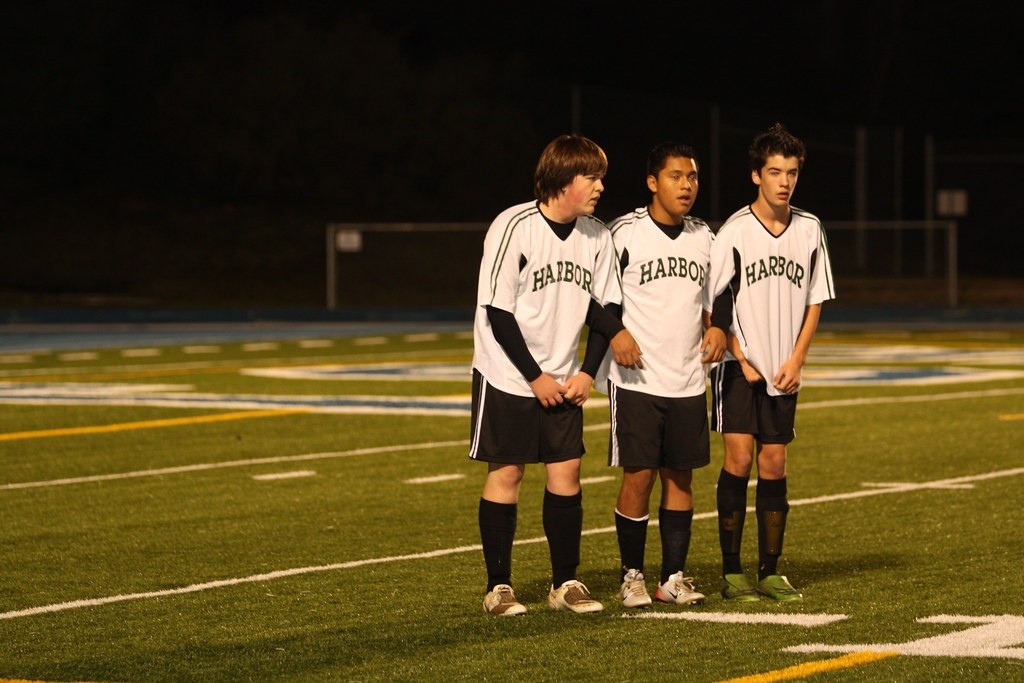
xmin=482 ymin=583 xmax=527 ymax=616
xmin=549 ymin=579 xmax=605 ymax=614
xmin=754 ymin=573 xmax=804 ymax=602
xmin=719 ymin=573 xmax=759 ymax=602
xmin=620 ymin=566 xmax=652 ymax=607
xmin=654 ymin=571 xmax=706 ymax=605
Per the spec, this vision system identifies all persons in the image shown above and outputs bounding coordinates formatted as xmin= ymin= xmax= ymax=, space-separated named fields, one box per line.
xmin=701 ymin=122 xmax=835 ymax=602
xmin=467 ymin=133 xmax=624 ymax=617
xmin=584 ymin=140 xmax=733 ymax=607
